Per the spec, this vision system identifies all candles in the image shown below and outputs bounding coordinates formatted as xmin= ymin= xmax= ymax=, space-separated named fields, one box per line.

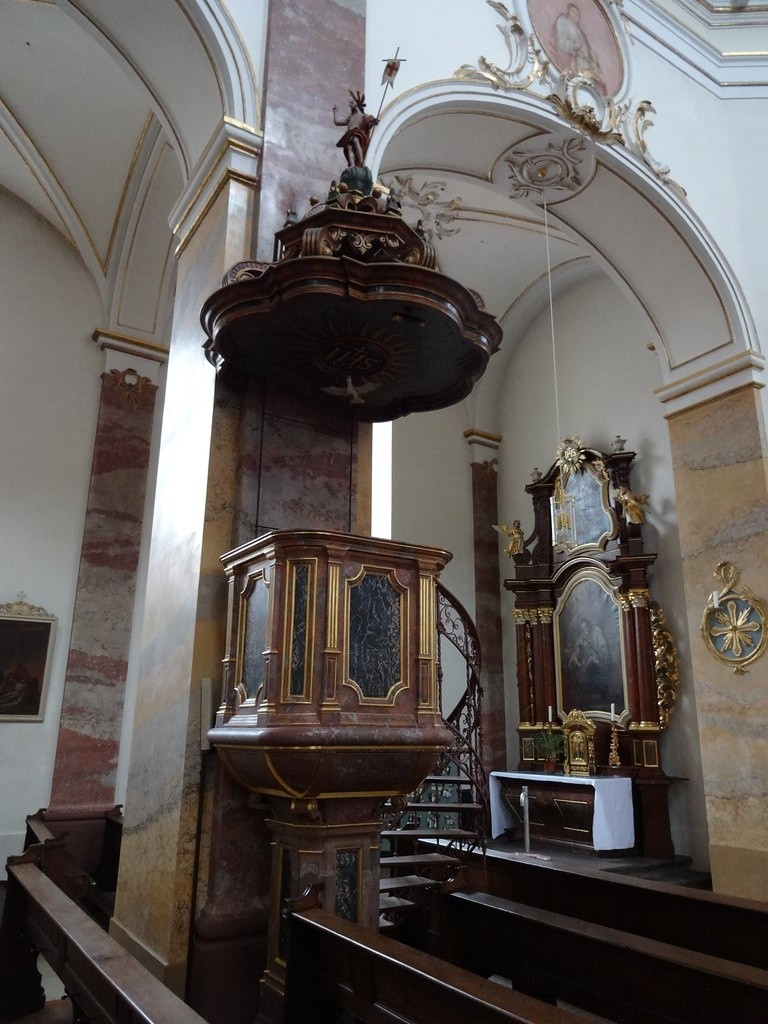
xmin=548 ymin=705 xmax=552 ymax=722
xmin=611 ymin=703 xmax=616 ymax=721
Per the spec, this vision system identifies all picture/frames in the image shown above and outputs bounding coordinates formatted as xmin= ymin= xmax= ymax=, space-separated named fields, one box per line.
xmin=633 ymin=740 xmax=641 ymax=765
xmin=514 ymin=0 xmax=637 ymax=107
xmin=522 ymin=738 xmax=535 ymax=761
xmin=0 ymin=600 xmax=59 ymax=723
xmin=560 ymin=708 xmax=598 ymax=777
xmin=537 ymin=738 xmax=548 ymax=761
xmin=643 ymin=740 xmax=659 ymax=767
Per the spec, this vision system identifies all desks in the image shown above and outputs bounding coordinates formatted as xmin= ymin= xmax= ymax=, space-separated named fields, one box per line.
xmin=487 ymin=770 xmax=634 ymax=854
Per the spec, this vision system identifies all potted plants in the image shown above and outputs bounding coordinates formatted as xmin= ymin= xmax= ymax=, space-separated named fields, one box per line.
xmin=530 ymin=730 xmax=565 ymax=773
